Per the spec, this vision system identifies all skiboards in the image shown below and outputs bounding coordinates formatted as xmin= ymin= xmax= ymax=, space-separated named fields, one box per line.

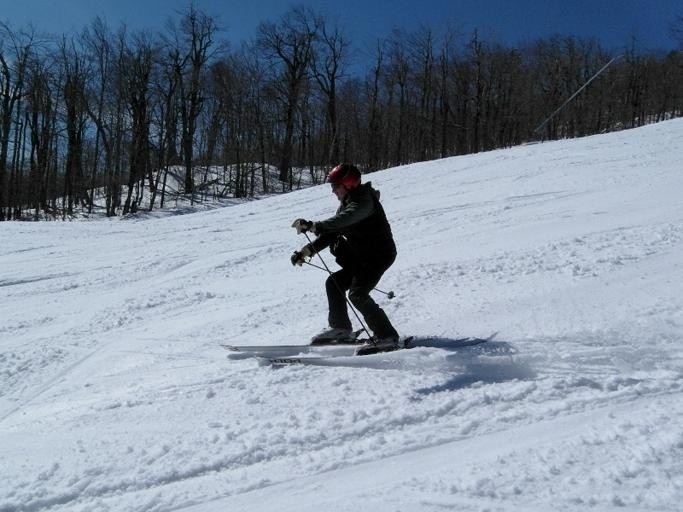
xmin=220 ymin=333 xmax=501 ymax=362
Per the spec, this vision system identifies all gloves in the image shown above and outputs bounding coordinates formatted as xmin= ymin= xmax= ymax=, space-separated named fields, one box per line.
xmin=290 ymin=247 xmax=311 ymax=267
xmin=291 ymin=218 xmax=316 ymax=235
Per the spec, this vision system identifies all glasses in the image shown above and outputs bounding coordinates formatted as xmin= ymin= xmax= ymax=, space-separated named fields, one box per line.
xmin=330 ymin=183 xmax=342 ymax=189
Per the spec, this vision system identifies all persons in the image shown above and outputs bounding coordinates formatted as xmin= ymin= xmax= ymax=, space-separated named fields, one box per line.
xmin=289 ymin=161 xmax=399 ymax=347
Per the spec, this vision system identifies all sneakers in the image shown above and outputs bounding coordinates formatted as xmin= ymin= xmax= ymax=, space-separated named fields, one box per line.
xmin=355 ymin=333 xmax=399 ymax=352
xmin=311 ymin=326 xmax=352 ymax=341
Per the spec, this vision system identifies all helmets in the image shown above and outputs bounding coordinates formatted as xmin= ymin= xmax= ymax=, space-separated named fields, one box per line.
xmin=326 ymin=163 xmax=361 ymax=190
